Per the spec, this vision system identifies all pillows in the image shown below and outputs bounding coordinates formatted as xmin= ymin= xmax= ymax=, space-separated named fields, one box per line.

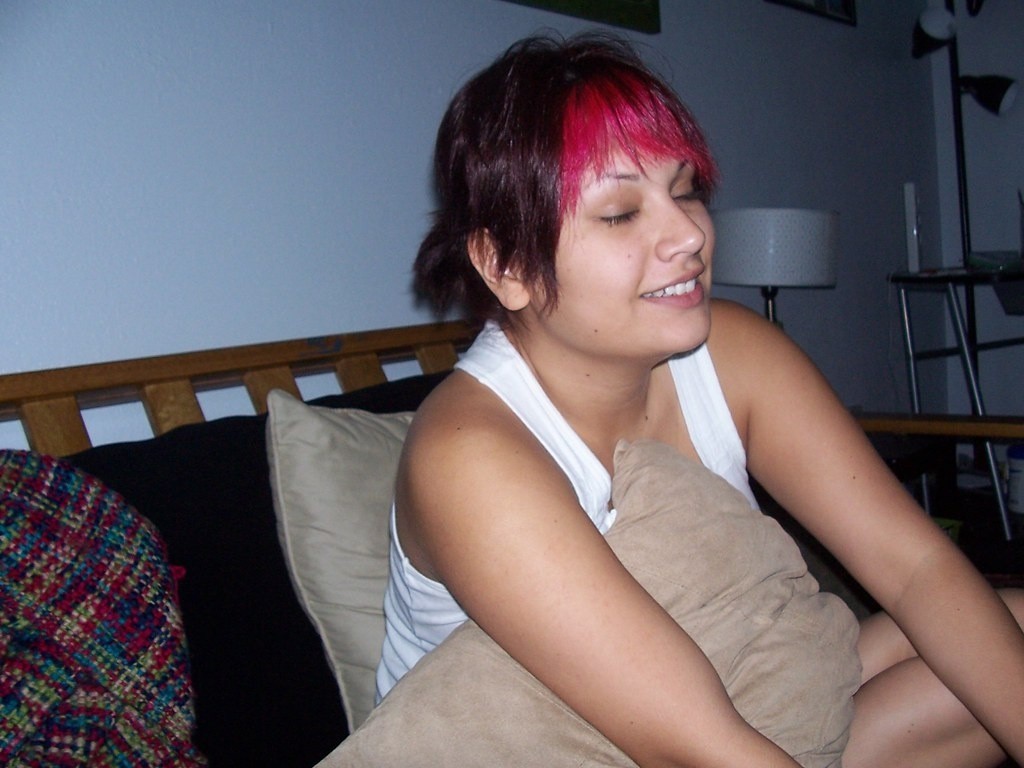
xmin=313 ymin=435 xmax=863 ymax=768
xmin=257 ymin=386 xmax=417 ymax=734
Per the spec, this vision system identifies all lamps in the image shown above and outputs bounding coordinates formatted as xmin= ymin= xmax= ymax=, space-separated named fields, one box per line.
xmin=911 ymin=0 xmax=1018 ymax=471
xmin=710 ymin=205 xmax=843 ymax=326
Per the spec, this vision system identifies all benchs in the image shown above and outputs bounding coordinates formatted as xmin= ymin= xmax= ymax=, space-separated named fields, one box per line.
xmin=0 ymin=319 xmax=1024 ymax=768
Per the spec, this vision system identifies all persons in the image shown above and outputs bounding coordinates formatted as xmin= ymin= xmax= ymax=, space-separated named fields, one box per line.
xmin=371 ymin=25 xmax=1024 ymax=768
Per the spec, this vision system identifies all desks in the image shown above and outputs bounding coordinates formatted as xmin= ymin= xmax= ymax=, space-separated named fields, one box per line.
xmin=890 ymin=270 xmax=1024 ymax=542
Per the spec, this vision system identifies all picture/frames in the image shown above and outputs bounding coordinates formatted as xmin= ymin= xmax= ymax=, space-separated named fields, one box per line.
xmin=766 ymin=0 xmax=857 ymax=26
